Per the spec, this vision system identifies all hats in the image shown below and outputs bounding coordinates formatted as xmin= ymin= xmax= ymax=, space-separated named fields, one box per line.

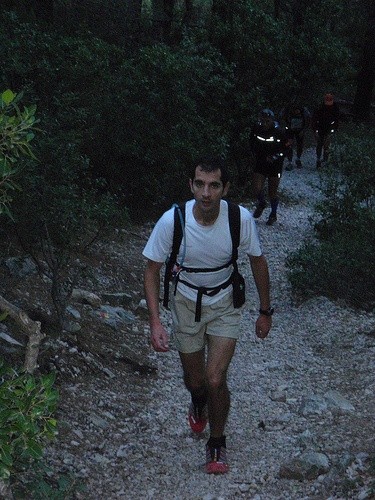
xmin=323 ymin=93 xmax=333 ymax=101
xmin=260 ymin=108 xmax=274 ymax=117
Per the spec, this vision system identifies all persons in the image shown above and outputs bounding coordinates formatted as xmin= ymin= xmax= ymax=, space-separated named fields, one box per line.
xmin=278 ymin=94 xmax=340 ymax=170
xmin=142 ymin=154 xmax=274 ymax=472
xmin=249 ymin=110 xmax=292 ymax=224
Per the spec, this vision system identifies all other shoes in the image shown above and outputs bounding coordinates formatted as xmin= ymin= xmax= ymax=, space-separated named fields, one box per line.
xmin=266 ymin=214 xmax=277 ymax=225
xmin=253 ymin=201 xmax=267 ymax=219
xmin=296 ymin=160 xmax=301 ymax=168
xmin=286 ymin=164 xmax=293 ymax=170
xmin=323 ymin=153 xmax=328 ymax=161
xmin=316 ymin=161 xmax=321 ymax=168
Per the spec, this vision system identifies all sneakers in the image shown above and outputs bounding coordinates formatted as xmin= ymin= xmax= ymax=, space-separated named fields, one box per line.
xmin=205 ymin=440 xmax=228 ymax=474
xmin=188 ymin=399 xmax=208 ymax=433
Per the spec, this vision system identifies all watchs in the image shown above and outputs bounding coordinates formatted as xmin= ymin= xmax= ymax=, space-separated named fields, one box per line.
xmin=259 ymin=306 xmax=274 ymax=317
